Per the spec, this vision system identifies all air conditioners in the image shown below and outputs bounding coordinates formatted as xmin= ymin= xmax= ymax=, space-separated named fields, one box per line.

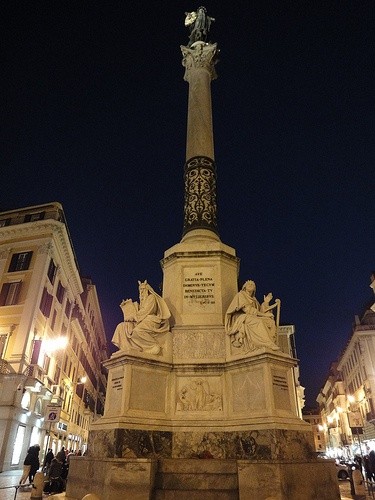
xmin=52 ymin=384 xmax=65 ymax=401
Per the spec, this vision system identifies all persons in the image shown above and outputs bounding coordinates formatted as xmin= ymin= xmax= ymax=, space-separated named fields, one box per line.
xmin=18 ymin=444 xmax=83 ymax=494
xmin=187 ymin=6 xmax=212 ymax=50
xmin=230 ymin=279 xmax=281 ymax=350
xmin=353 ymin=450 xmax=375 ymax=482
xmin=111 ymin=279 xmax=172 ymax=355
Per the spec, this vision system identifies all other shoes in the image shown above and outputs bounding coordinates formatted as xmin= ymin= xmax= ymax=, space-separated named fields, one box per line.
xmin=369 ymin=480 xmax=374 ymax=484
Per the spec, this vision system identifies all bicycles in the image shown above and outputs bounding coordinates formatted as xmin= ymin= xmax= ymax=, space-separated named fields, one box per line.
xmin=48 ymin=476 xmax=59 ymax=496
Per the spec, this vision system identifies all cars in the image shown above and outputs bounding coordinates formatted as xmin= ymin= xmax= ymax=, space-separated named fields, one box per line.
xmin=335 ymin=462 xmax=349 ymax=480
